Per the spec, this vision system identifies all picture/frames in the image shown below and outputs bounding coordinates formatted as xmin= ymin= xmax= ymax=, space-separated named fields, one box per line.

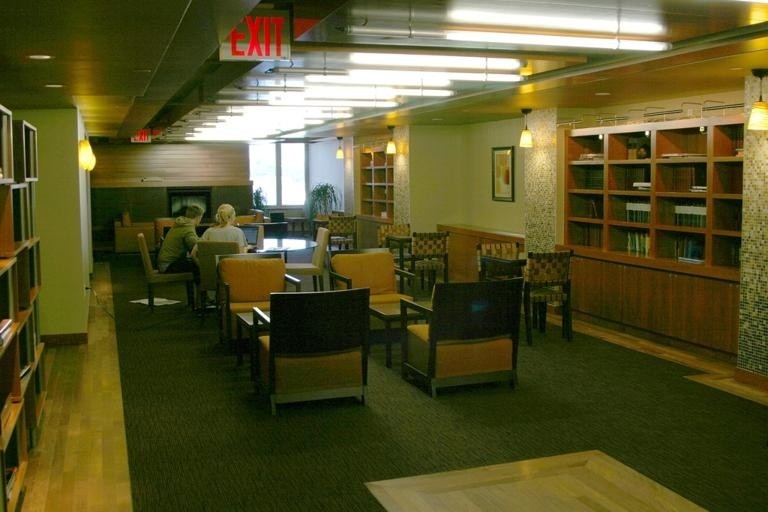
xmin=492 ymin=146 xmax=514 ymax=202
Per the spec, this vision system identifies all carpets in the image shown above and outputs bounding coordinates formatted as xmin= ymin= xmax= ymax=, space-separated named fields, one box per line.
xmin=109 ymin=256 xmax=767 ymax=512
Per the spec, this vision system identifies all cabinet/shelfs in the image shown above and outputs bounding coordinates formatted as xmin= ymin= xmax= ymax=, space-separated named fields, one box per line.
xmin=353 ymin=139 xmax=394 ymax=225
xmin=0 ymin=104 xmax=47 ymax=511
xmin=555 ymin=116 xmax=744 ymax=355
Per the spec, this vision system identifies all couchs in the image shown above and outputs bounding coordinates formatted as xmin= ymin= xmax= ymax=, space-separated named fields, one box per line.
xmin=236 ymin=209 xmax=264 ymax=224
xmin=115 ymin=213 xmax=155 ymax=252
xmin=155 ymin=218 xmax=175 ymax=240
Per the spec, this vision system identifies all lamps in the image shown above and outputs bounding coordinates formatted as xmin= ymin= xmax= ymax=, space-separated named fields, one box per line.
xmin=185 ymin=107 xmax=353 ymax=145
xmin=301 ymin=86 xmax=453 ymax=99
xmin=305 ymin=75 xmax=450 ymax=86
xmin=748 ymin=69 xmax=768 ymax=130
xmin=446 ymin=11 xmax=667 ymax=35
xmin=441 ymin=31 xmax=671 ymax=51
xmin=347 ymin=71 xmax=524 ymax=83
xmin=350 ymin=52 xmax=520 ymax=72
xmin=80 ymin=137 xmax=96 ymax=171
xmin=336 ymin=137 xmax=344 ymax=159
xmin=519 ymin=109 xmax=533 ymax=148
xmin=386 ymin=126 xmax=396 ymax=154
xmin=269 ymin=98 xmax=398 ymax=109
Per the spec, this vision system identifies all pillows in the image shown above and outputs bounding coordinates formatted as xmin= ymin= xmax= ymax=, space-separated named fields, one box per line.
xmin=122 ymin=212 xmax=131 ymax=227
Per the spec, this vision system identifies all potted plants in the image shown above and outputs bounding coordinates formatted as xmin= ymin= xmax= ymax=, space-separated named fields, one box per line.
xmin=308 ymin=184 xmax=338 ymax=220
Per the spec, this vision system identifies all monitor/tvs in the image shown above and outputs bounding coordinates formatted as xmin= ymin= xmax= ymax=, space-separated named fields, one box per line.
xmin=232 ymin=223 xmax=264 ymax=250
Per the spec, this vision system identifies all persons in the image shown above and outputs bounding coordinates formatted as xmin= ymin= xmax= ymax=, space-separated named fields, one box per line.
xmin=156 ymin=204 xmax=205 ymax=287
xmin=190 ymin=203 xmax=248 ymax=267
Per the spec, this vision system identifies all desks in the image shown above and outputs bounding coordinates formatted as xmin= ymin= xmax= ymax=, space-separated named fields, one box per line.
xmin=286 ymin=217 xmax=308 ymax=231
xmin=480 ymin=244 xmax=574 ymax=333
xmin=236 ymin=311 xmax=270 ymax=381
xmin=369 ymin=303 xmax=427 ymax=368
xmin=243 ymin=239 xmax=319 ymax=263
xmin=386 ymin=235 xmax=436 ymax=294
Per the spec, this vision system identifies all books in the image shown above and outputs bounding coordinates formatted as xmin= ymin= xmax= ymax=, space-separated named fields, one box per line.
xmin=632 ymin=182 xmax=652 ymax=192
xmin=625 ymin=202 xmax=650 ymax=225
xmin=688 ymin=185 xmax=708 ymax=194
xmin=672 ymin=234 xmax=705 ymax=263
xmin=625 ymin=231 xmax=648 ymax=256
xmin=675 ymin=206 xmax=706 ymax=229
xmin=0 ymin=316 xmax=15 ymax=344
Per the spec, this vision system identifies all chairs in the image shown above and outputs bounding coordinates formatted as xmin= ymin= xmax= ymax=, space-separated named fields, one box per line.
xmin=328 ymin=252 xmax=428 ymax=304
xmin=138 ymin=233 xmax=194 ymax=313
xmin=523 ymin=251 xmax=570 ymax=345
xmin=270 ymin=213 xmax=284 ymax=222
xmin=285 ymin=227 xmax=330 ymax=291
xmin=198 ymin=242 xmax=239 ymax=317
xmin=253 ymin=288 xmax=371 ymax=416
xmin=399 ymin=278 xmax=524 ymax=397
xmin=476 ymin=241 xmax=522 ymax=280
xmin=412 ymin=232 xmax=449 ymax=293
xmin=328 ymin=215 xmax=357 ymax=251
xmin=312 ymin=221 xmax=349 ymax=250
xmin=216 ymin=253 xmax=301 ymax=351
xmin=377 ymin=224 xmax=425 ymax=290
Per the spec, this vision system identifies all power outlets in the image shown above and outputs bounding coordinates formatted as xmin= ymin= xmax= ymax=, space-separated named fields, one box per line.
xmin=84 ymin=285 xmax=90 ymax=296
